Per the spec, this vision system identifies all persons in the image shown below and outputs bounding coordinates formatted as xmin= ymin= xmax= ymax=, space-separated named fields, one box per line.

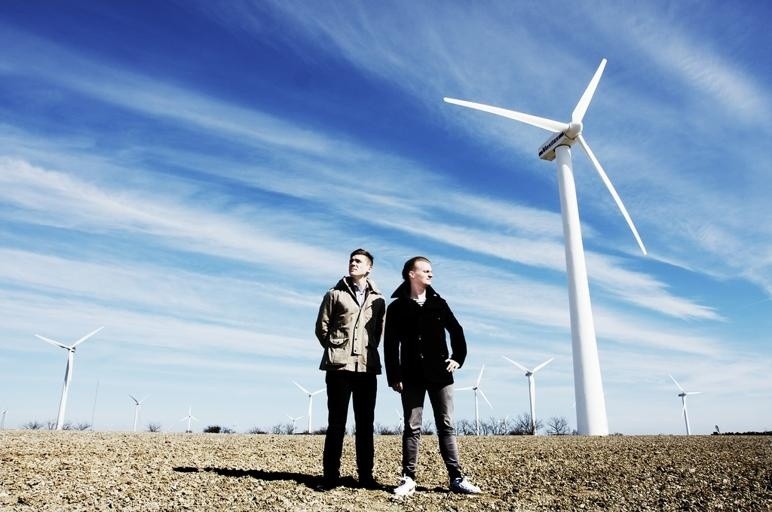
xmin=315 ymin=248 xmax=387 ymax=493
xmin=385 ymin=255 xmax=485 ymax=498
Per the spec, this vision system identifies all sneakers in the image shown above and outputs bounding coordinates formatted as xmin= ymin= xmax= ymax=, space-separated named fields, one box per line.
xmin=394 ymin=474 xmax=418 ymax=495
xmin=452 ymin=476 xmax=481 ymax=493
